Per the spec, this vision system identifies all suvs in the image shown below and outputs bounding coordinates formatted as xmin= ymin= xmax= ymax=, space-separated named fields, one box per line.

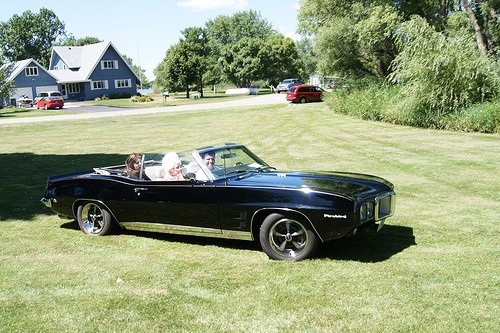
xmin=276 ymin=79 xmax=300 ymax=93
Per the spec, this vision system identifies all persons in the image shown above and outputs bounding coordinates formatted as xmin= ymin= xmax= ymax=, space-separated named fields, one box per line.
xmin=161 ymin=152 xmax=185 ymax=181
xmin=196 ymin=152 xmax=222 ymax=181
xmin=125 ymin=153 xmax=151 ymax=180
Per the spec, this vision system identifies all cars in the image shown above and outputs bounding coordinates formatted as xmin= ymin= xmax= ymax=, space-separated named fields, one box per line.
xmin=40 ymin=141 xmax=398 ymax=262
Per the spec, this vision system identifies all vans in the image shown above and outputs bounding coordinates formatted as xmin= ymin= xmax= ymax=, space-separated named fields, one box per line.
xmin=286 ymin=83 xmax=329 ymax=103
xmin=34 ymin=90 xmax=64 ymax=110
